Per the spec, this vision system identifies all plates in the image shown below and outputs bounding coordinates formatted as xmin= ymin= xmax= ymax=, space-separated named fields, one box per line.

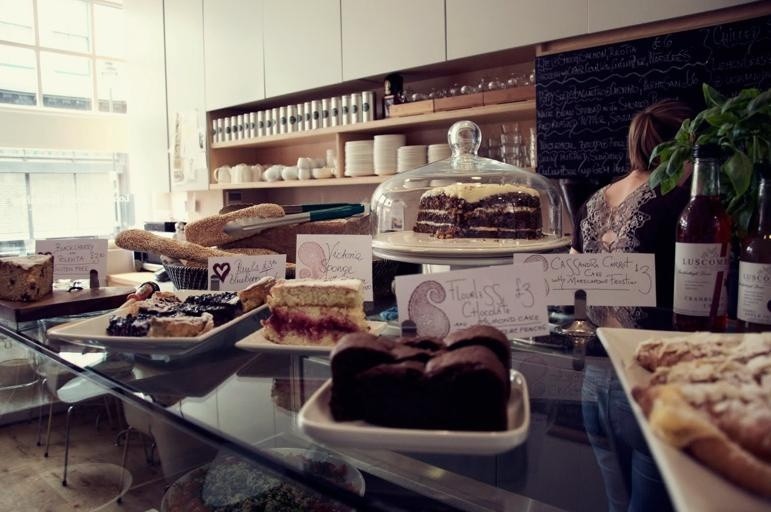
xmin=39 ymin=286 xmax=270 ymax=355
xmin=226 ymin=351 xmax=342 ymax=380
xmin=342 ymin=133 xmax=451 ymax=176
xmin=12 ymin=461 xmax=133 ymax=510
xmin=594 ymin=327 xmax=771 ymax=509
xmin=300 ymin=370 xmax=533 ymax=461
xmin=233 ymin=314 xmax=389 ymax=361
xmin=57 ymin=344 xmax=260 ymax=405
xmin=158 ymin=444 xmax=366 ymax=510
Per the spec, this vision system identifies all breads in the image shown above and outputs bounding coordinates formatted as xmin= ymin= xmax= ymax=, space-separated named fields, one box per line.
xmin=106 ymin=276 xmax=275 ymax=337
xmin=115 ymin=203 xmax=296 ymax=270
xmin=631 ymin=330 xmax=771 ymax=498
xmin=328 ymin=322 xmax=513 ymax=431
xmin=261 ymin=279 xmax=370 ymax=350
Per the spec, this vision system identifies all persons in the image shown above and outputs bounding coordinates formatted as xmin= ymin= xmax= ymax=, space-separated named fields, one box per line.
xmin=569 ymin=97 xmax=698 ymax=511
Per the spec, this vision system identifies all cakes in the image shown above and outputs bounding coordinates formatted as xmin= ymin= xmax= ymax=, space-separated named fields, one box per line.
xmin=0 ymin=252 xmax=55 ymax=304
xmin=413 ymin=182 xmax=542 ymax=241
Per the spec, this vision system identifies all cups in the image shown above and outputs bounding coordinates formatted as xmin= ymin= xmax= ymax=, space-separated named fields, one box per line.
xmin=209 ymin=150 xmax=343 ymax=183
xmin=480 ymin=122 xmax=534 ymax=169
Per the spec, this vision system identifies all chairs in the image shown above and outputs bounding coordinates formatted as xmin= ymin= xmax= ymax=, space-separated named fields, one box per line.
xmin=36 ymin=361 xmax=170 ymax=504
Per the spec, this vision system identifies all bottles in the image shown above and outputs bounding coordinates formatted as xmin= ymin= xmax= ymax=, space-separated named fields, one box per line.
xmin=737 ymin=162 xmax=769 ymax=331
xmin=384 ymin=66 xmax=539 ymax=102
xmin=211 ymin=89 xmax=373 ymax=145
xmin=675 ymin=141 xmax=735 ymax=325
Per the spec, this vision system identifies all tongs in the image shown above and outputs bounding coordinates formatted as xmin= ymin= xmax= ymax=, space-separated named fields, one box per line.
xmin=218 ymin=200 xmax=366 ymax=238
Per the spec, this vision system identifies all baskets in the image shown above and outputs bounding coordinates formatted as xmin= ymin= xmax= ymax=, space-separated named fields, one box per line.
xmin=162 ymin=264 xmax=296 ymax=291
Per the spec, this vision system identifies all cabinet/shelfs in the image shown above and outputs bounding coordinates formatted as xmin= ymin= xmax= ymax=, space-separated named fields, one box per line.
xmin=205 ymin=42 xmax=537 ymax=191
